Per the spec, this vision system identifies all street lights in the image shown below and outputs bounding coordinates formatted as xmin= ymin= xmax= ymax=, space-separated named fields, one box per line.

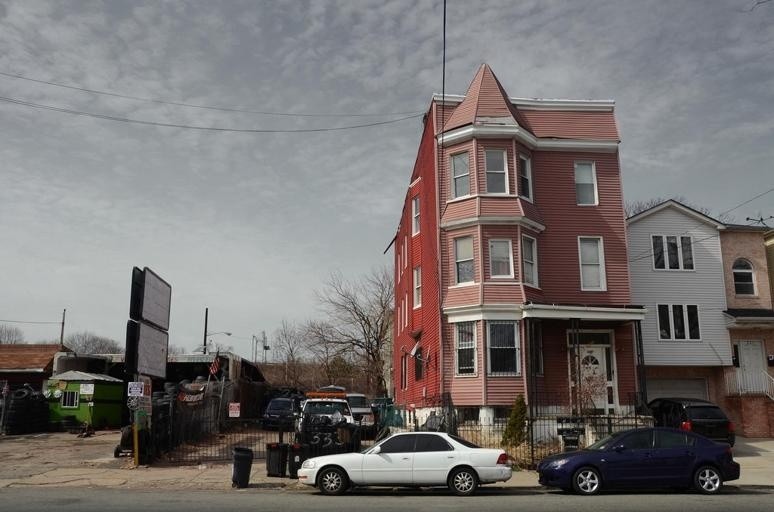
xmin=203 ymin=306 xmax=231 ymax=354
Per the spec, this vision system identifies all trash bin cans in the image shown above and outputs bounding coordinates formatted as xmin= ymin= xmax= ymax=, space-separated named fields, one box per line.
xmin=265 ymin=443 xmax=288 ymax=477
xmin=288 ymin=444 xmax=310 ymax=479
xmin=231 ymin=447 xmax=253 ymax=488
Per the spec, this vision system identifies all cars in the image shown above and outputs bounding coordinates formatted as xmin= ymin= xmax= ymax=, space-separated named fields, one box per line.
xmin=639 ymin=396 xmax=736 ymax=448
xmin=297 ymin=431 xmax=512 ymax=495
xmin=263 ymin=384 xmax=378 ymax=455
xmin=537 ymin=427 xmax=741 ymax=495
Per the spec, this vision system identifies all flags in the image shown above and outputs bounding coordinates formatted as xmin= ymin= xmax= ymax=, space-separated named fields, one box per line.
xmin=209 ymin=349 xmax=220 ymax=376
